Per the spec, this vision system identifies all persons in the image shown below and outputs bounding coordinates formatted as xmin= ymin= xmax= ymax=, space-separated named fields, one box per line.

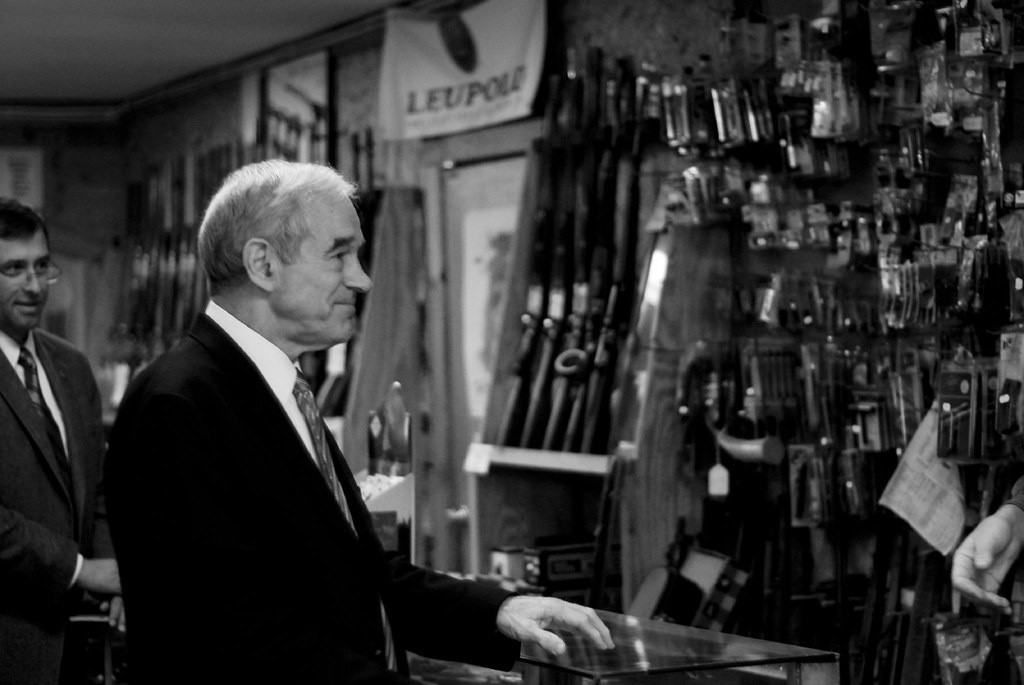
xmin=951 ymin=474 xmax=1024 ymax=617
xmin=0 ymin=194 xmax=128 ymax=684
xmin=101 ymin=160 xmax=615 ymax=685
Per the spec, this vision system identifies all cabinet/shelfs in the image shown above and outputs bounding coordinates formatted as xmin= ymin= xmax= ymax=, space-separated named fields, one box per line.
xmin=407 ymin=605 xmax=842 ymax=684
xmin=313 ymin=131 xmax=733 ymax=618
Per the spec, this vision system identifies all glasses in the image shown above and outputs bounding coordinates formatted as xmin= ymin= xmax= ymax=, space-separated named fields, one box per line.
xmin=0 ymin=259 xmax=62 ymax=286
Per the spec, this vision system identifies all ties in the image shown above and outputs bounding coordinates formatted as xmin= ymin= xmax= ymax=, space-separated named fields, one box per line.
xmin=19 ymin=345 xmax=77 ymax=500
xmin=294 ymin=367 xmax=396 ymax=672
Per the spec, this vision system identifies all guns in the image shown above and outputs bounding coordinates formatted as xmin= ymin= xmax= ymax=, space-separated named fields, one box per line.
xmin=490 ymin=44 xmax=659 ymax=458
xmin=107 ymin=124 xmax=381 ymax=414
